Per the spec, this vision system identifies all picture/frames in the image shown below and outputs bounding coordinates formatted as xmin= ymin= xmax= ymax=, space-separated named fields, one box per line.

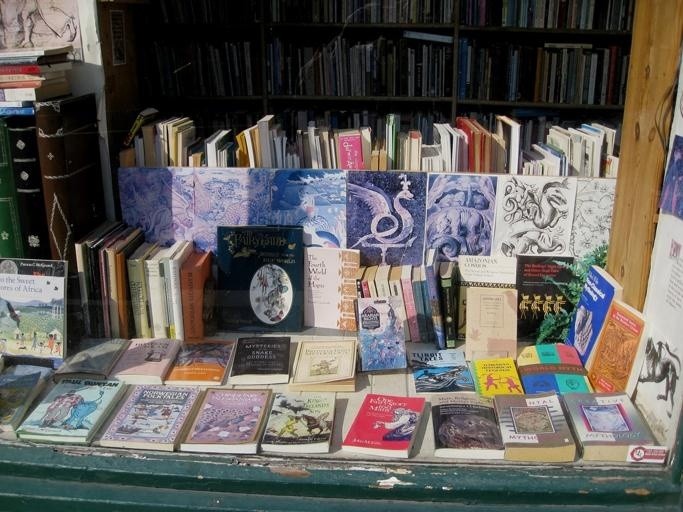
xmin=110 ymin=8 xmax=127 ymax=66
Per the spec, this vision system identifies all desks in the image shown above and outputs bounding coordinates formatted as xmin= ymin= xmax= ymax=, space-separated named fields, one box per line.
xmin=0 ymin=409 xmax=682 ymax=511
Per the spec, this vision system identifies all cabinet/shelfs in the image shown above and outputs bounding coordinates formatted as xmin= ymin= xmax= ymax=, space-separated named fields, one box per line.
xmin=137 ymin=0 xmax=635 ymax=126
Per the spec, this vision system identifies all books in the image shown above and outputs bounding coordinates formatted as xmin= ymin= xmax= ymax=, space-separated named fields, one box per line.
xmin=0 ymin=1 xmax=682 ymax=464
